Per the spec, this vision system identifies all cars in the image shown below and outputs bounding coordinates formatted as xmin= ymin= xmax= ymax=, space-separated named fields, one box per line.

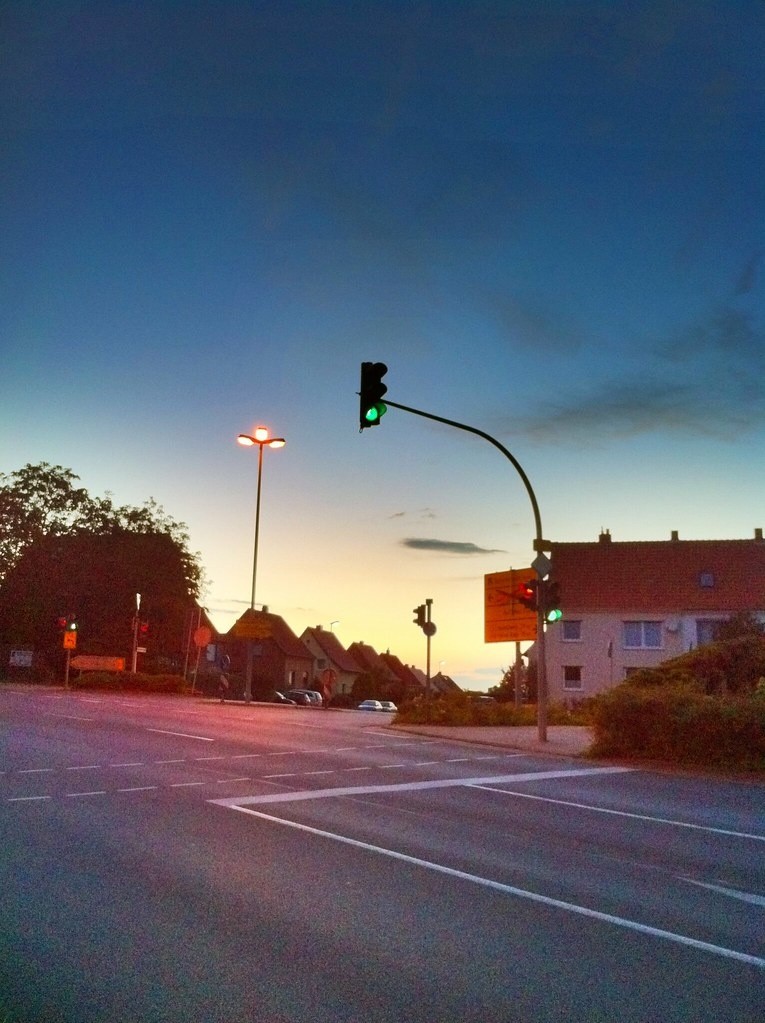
xmin=274 ymin=687 xmax=322 ymax=704
xmin=357 ymin=700 xmax=399 ymax=714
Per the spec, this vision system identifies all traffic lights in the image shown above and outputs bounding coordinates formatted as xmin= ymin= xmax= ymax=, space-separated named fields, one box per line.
xmin=139 ymin=620 xmax=150 ymax=636
xmin=543 ymin=579 xmax=560 ymax=626
xmin=412 ymin=603 xmax=427 ymax=627
xmin=57 ymin=615 xmax=67 ymax=631
xmin=358 ymin=359 xmax=389 ymax=431
xmin=518 ymin=578 xmax=540 ymax=613
xmin=68 ymin=614 xmax=79 ymax=633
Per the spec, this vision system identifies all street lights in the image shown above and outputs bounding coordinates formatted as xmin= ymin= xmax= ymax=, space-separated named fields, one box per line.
xmin=233 ymin=421 xmax=288 ymax=704
